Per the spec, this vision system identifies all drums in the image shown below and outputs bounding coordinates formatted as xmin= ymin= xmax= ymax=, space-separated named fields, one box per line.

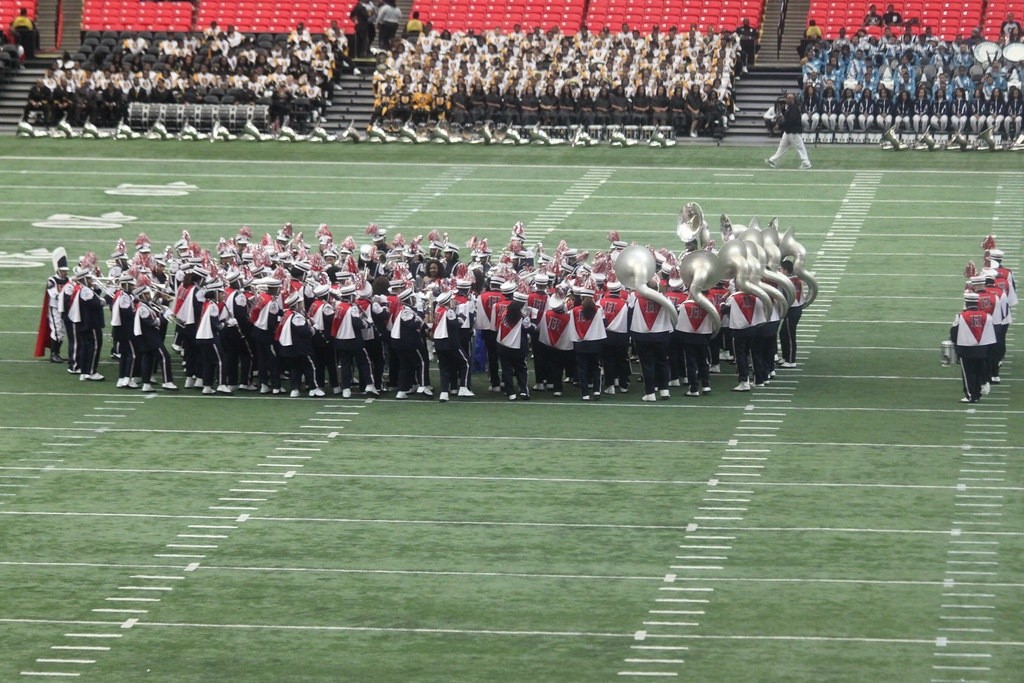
xmin=939 ymin=340 xmax=960 ymax=365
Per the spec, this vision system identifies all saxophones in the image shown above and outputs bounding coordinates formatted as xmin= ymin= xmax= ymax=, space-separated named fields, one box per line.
xmin=418 ymin=296 xmax=434 ymax=342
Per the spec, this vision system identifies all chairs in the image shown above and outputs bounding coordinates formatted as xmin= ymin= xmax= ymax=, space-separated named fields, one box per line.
xmin=0 ymin=0 xmax=37 ymax=77
xmin=81 ymin=0 xmax=359 ymax=104
xmin=806 ymin=0 xmax=1024 ymax=78
xmin=410 ymin=0 xmax=764 ymax=38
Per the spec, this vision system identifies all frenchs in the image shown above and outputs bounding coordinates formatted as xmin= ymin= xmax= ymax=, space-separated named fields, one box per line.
xmin=675 ymin=200 xmax=820 ymax=325
xmin=676 ymin=249 xmax=725 ymax=341
xmin=613 ymin=244 xmax=679 ymax=327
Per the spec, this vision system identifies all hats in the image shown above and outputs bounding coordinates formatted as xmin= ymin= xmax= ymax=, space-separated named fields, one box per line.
xmin=982 ymin=266 xmax=998 ymax=278
xmin=983 ymin=274 xmax=996 ymax=286
xmin=964 ymin=292 xmax=980 ymax=302
xmin=990 ymin=260 xmax=1000 ymax=270
xmin=970 ymin=276 xmax=986 ymax=285
xmin=46 ymin=221 xmax=736 ymax=303
xmin=989 ymin=249 xmax=1004 ymax=259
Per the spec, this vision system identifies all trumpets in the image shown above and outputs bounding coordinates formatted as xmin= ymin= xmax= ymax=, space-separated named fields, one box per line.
xmin=69 ymin=259 xmax=188 ymax=329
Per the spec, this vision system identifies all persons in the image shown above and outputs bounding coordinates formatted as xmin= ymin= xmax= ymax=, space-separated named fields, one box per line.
xmin=349 ymin=0 xmax=402 ymax=58
xmin=949 ymin=250 xmax=1018 ymax=404
xmin=0 ymin=8 xmax=41 ymax=70
xmin=366 ymin=12 xmax=761 ymax=138
xmin=34 ymin=222 xmax=807 ymax=404
xmin=22 ymin=21 xmax=361 ymax=128
xmin=763 ymin=4 xmax=1024 ymax=169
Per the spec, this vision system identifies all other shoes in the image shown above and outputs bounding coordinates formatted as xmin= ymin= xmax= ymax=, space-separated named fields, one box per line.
xmin=960 ymin=397 xmax=970 ymax=403
xmin=991 ymin=376 xmax=1000 ymax=383
xmin=764 ymin=158 xmax=774 ymax=167
xmin=50 ymin=343 xmax=799 ymax=402
xmin=799 ymin=162 xmax=811 ymax=169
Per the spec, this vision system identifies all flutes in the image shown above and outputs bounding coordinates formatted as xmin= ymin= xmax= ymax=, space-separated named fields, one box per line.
xmin=222 ymin=300 xmax=255 ymax=358
xmin=301 ymin=307 xmax=331 ymax=345
xmin=355 ymin=300 xmax=383 ymax=338
